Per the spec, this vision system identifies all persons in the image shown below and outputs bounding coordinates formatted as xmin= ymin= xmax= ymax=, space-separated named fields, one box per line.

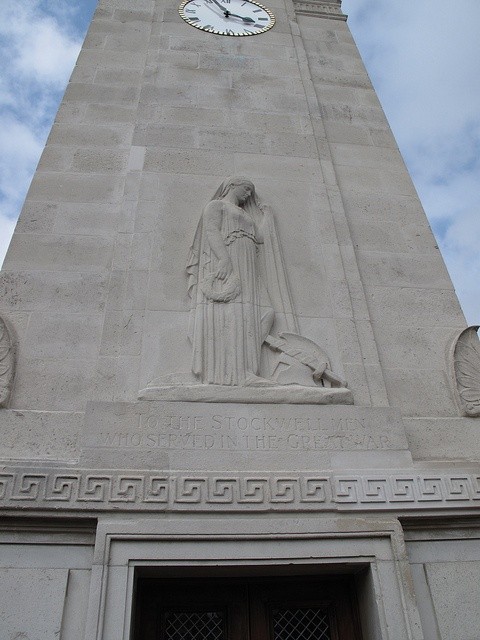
xmin=187 ymin=175 xmax=299 ymax=387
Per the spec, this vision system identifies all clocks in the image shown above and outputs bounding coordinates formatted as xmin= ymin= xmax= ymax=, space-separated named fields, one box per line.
xmin=177 ymin=0 xmax=276 ymax=37
